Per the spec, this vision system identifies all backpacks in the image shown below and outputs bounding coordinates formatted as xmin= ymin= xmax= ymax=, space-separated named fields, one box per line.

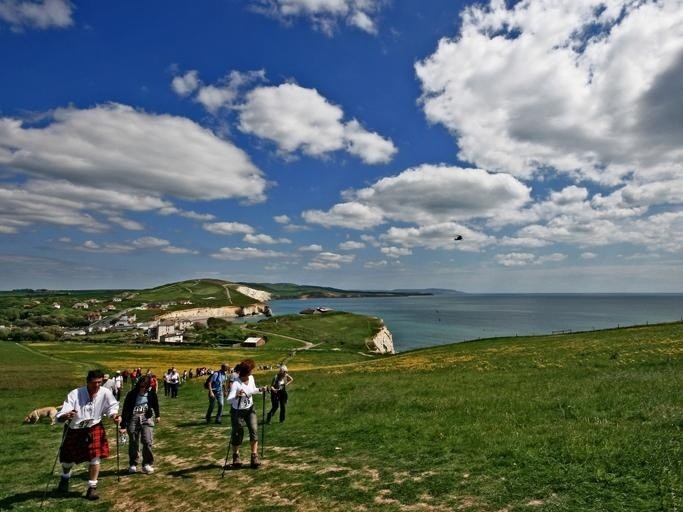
xmin=205 ymin=372 xmax=219 ymax=389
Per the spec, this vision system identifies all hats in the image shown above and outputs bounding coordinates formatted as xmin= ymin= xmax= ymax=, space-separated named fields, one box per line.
xmin=104 ymin=374 xmax=109 ymax=380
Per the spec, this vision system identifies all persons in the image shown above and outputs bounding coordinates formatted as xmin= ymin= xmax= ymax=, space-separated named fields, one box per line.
xmin=100 ymin=373 xmax=114 ymax=416
xmin=118 ymin=375 xmax=160 ymax=476
xmin=54 ymin=368 xmax=122 ymax=501
xmin=111 ymin=363 xmax=273 ymax=402
xmin=225 ymin=359 xmax=269 ymax=468
xmin=264 ymin=365 xmax=294 ymax=425
xmin=203 ymin=362 xmax=229 ymax=425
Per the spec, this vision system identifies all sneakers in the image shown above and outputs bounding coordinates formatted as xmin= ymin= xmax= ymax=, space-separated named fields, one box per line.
xmin=87 ymin=488 xmax=100 ymax=499
xmin=142 ymin=465 xmax=153 ymax=472
xmin=58 ymin=478 xmax=68 ymax=496
xmin=129 ymin=465 xmax=136 ymax=473
xmin=205 ymin=417 xmax=221 ymax=424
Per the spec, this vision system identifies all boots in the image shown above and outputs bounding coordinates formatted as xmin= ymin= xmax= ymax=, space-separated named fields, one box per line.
xmin=232 ymin=453 xmax=243 ymax=466
xmin=251 ymin=453 xmax=261 ymax=467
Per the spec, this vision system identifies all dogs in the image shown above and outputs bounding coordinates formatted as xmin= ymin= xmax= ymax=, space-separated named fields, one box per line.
xmin=24 ymin=404 xmax=63 ymax=427
